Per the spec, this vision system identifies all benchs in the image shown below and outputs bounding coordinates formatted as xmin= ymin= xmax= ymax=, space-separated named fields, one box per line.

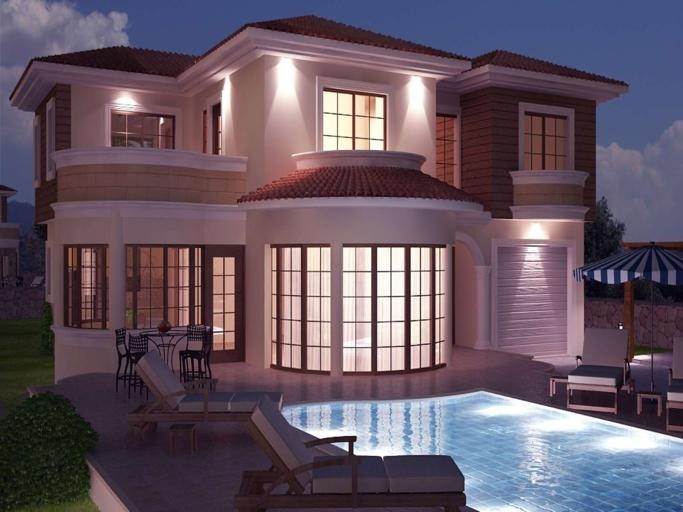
xmin=549 ymin=375 xmax=633 ymax=398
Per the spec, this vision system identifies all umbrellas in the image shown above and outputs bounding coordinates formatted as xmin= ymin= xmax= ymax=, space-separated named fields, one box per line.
xmin=574 ymin=240 xmax=683 ymax=402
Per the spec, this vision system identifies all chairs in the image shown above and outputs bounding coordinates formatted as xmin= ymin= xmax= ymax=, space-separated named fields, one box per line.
xmin=14 ymin=271 xmax=45 ymax=300
xmin=127 ymin=350 xmax=283 ymax=440
xmin=115 ymin=324 xmax=213 ymax=399
xmin=665 ymin=336 xmax=683 ymax=433
xmin=233 ymin=393 xmax=466 ymax=512
xmin=566 ymin=328 xmax=633 ymax=416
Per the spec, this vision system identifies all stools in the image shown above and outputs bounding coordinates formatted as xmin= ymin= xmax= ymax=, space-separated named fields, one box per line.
xmin=168 ymin=424 xmax=198 ymax=456
xmin=637 ymin=391 xmax=662 ymax=417
